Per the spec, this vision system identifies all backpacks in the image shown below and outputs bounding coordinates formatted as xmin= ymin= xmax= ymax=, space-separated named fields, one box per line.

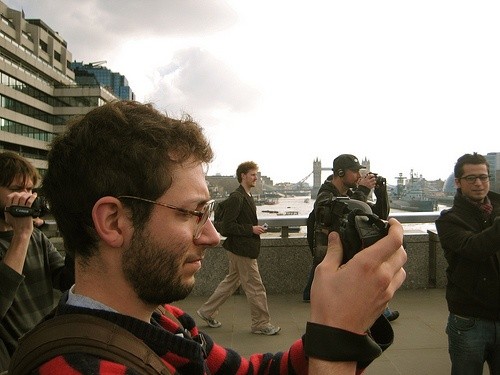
xmin=212 ymin=191 xmax=257 ymax=233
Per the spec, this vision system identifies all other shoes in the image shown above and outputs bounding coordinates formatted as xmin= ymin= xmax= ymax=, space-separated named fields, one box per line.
xmin=303 ymin=295 xmax=310 ymax=300
xmin=388 ymin=311 xmax=399 ymax=321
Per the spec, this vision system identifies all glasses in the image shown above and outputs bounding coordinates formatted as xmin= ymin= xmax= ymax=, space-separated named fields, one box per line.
xmin=116 ymin=196 xmax=217 ymax=240
xmin=458 ymin=174 xmax=491 ymax=184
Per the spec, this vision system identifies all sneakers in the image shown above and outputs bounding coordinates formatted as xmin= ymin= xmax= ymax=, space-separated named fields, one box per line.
xmin=196 ymin=309 xmax=221 ymax=328
xmin=253 ymin=323 xmax=281 ymax=335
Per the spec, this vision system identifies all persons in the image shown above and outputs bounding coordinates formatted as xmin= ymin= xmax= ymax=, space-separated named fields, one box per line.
xmin=303 ymin=154 xmax=399 ymax=321
xmin=435 ymin=152 xmax=500 ymax=375
xmin=196 ymin=162 xmax=281 ymax=335
xmin=10 ymin=101 xmax=407 ymax=375
xmin=0 ymin=150 xmax=72 ymax=375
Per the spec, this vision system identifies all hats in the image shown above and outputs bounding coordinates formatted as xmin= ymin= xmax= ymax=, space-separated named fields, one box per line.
xmin=333 ymin=154 xmax=366 ymax=172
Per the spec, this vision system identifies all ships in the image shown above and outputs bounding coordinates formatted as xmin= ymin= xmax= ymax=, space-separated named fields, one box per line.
xmin=386 ymin=168 xmax=439 ymax=212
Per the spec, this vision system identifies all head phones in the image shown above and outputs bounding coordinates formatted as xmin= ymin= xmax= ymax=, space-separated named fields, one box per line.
xmin=333 ymin=160 xmax=345 ymax=177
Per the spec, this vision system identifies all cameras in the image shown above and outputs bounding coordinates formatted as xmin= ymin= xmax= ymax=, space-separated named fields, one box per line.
xmin=26 ymin=195 xmax=48 ymax=218
xmin=365 ymin=172 xmax=387 ymax=195
xmin=338 ymin=213 xmax=391 ymax=266
